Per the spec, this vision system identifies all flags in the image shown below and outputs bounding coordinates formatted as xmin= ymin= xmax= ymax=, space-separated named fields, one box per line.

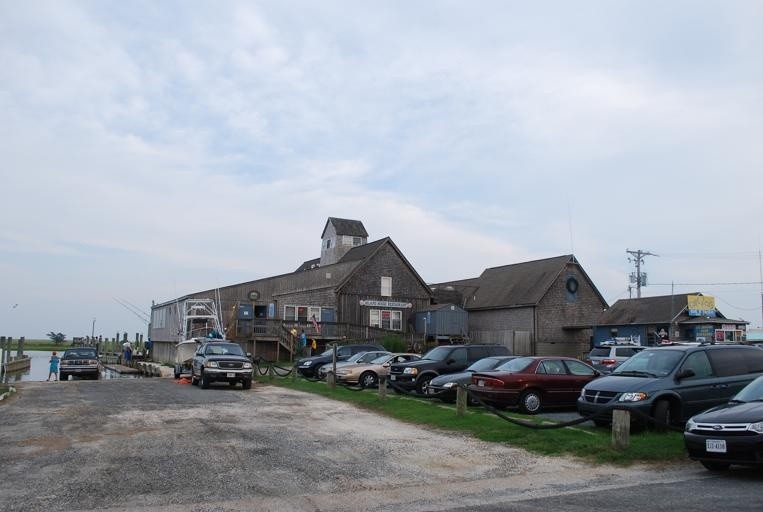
xmin=310 ymin=314 xmax=320 ymax=334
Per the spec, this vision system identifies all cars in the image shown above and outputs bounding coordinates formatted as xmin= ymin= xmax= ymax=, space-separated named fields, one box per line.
xmin=59 ymin=347 xmax=103 ymax=381
xmin=190 ymin=340 xmax=255 ymax=390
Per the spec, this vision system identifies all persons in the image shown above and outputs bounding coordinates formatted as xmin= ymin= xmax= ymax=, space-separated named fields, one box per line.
xmin=310 ymin=337 xmax=316 ymax=356
xmin=289 ymin=325 xmax=297 ymax=352
xmin=121 ymin=338 xmax=133 ymax=368
xmin=142 ymin=337 xmax=152 ymax=362
xmin=298 ymin=329 xmax=308 ymax=356
xmin=657 ymin=326 xmax=667 ymax=342
xmin=45 ymin=351 xmax=58 ymax=381
